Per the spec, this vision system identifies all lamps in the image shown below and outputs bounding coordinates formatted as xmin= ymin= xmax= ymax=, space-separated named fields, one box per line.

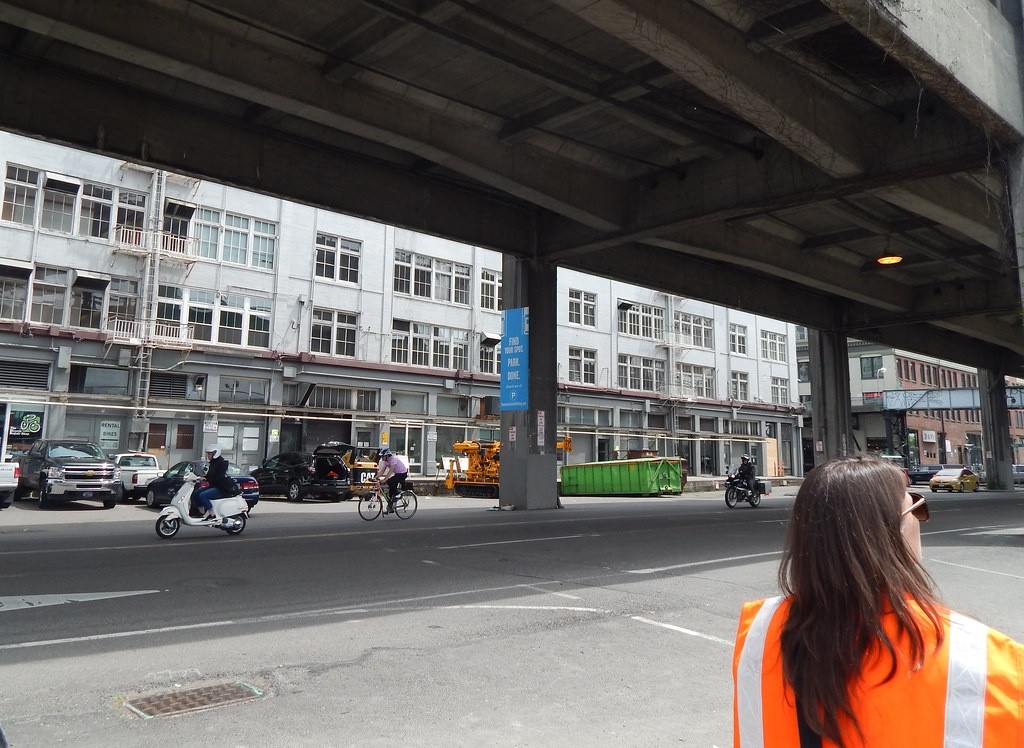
xmin=875 ymin=237 xmax=904 ymax=265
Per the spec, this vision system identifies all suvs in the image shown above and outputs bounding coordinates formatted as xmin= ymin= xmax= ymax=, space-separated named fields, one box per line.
xmin=248 ymin=442 xmax=356 ymax=502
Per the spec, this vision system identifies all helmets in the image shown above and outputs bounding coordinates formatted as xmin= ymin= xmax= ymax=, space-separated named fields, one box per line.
xmin=741 ymin=454 xmax=750 ymax=461
xmin=205 ymin=444 xmax=221 ymax=459
xmin=378 ymin=447 xmax=393 ymax=456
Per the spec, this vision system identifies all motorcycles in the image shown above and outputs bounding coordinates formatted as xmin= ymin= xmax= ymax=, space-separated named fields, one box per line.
xmin=723 ymin=464 xmax=772 ymax=508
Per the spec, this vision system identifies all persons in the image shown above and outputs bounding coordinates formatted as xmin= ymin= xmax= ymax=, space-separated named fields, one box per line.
xmin=738 ymin=454 xmax=757 ymax=498
xmin=192 ymin=444 xmax=226 ymax=521
xmin=375 ymin=448 xmax=408 ymax=515
xmin=733 ymin=454 xmax=1024 ymax=748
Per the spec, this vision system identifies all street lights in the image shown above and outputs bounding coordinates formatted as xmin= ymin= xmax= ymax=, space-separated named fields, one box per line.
xmin=877 ymin=367 xmax=887 ymax=401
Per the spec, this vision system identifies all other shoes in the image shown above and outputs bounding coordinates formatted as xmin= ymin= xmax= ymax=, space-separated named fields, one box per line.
xmin=195 ymin=513 xmax=204 ymax=518
xmin=754 ymin=495 xmax=757 ymax=498
xmin=394 ymin=494 xmax=402 ymax=500
xmin=202 ymin=514 xmax=216 ymax=521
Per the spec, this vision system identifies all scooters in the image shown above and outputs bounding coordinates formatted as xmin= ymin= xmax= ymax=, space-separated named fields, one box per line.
xmin=155 ymin=466 xmax=249 ymax=539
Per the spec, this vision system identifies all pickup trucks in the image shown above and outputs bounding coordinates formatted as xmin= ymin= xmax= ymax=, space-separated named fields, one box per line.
xmin=11 ymin=439 xmax=123 ymax=509
xmin=113 ymin=453 xmax=180 ymax=503
xmin=0 ymin=462 xmax=20 ymax=508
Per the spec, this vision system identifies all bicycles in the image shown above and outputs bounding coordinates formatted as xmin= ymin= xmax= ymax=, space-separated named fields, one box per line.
xmin=358 ymin=478 xmax=418 ymax=522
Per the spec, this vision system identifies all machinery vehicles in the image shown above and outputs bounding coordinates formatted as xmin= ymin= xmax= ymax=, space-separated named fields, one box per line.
xmin=445 ymin=436 xmax=573 ymax=499
xmin=341 ymin=446 xmax=383 ymax=500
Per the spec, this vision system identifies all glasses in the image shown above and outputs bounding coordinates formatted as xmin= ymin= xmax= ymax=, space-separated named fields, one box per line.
xmin=902 ymin=492 xmax=929 ymax=523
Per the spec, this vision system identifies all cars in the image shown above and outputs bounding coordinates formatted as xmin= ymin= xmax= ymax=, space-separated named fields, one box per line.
xmin=979 ymin=465 xmax=1024 ymax=484
xmin=908 ymin=464 xmax=967 ymax=485
xmin=929 ymin=468 xmax=979 ymax=493
xmin=146 ymin=460 xmax=260 ymax=515
